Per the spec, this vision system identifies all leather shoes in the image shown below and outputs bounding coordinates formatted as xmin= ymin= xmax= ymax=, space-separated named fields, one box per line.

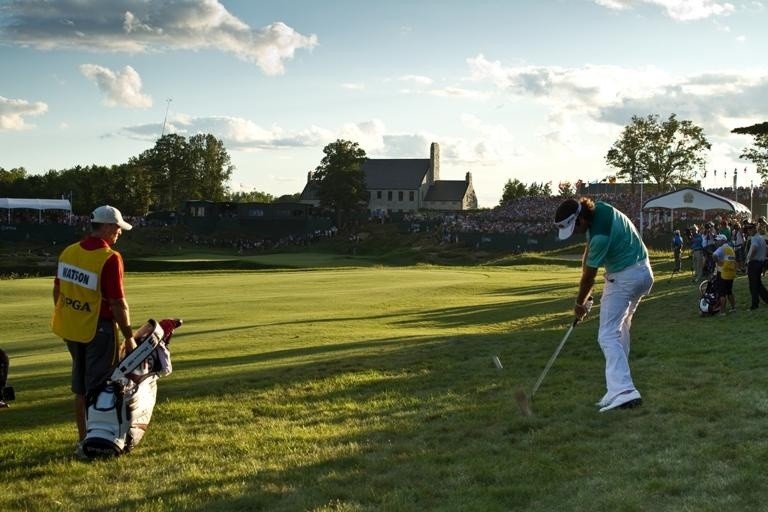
xmin=597 ymin=389 xmax=643 ymax=414
xmin=594 ymin=393 xmax=609 ymax=409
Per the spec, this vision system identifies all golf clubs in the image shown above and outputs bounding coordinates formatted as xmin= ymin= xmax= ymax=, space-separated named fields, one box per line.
xmin=514 ymin=298 xmax=593 ymax=415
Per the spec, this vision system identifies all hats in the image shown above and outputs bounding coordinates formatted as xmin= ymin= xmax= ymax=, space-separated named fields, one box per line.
xmin=673 ymin=229 xmax=680 ymax=235
xmin=714 ymin=233 xmax=728 ymax=242
xmin=90 ymin=204 xmax=133 ymax=232
xmin=553 ymin=201 xmax=583 ymax=241
xmin=744 ymin=224 xmax=758 ymax=230
xmin=687 ymin=225 xmax=698 ymax=231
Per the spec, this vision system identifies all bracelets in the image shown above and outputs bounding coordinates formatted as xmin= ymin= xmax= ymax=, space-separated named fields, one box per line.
xmin=575 ymin=302 xmax=585 ymax=308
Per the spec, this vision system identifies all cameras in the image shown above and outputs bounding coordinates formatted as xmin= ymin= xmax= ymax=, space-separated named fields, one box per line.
xmin=705 ymin=222 xmax=714 ymax=228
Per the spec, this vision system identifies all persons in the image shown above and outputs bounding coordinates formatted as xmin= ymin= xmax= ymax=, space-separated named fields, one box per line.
xmin=481 ymin=185 xmax=768 ymax=280
xmin=12 ymin=208 xmax=146 ymax=230
xmin=158 ymin=222 xmax=338 ymax=252
xmin=552 ymin=195 xmax=655 ymax=415
xmin=743 ymin=223 xmax=768 ymax=311
xmin=711 ymin=234 xmax=739 ymax=317
xmin=49 ymin=204 xmax=138 ymax=463
xmin=348 ymin=230 xmax=360 ymax=242
xmin=0 ymin=345 xmax=10 ymax=408
xmin=401 ymin=209 xmax=481 ymax=244
xmin=369 ymin=209 xmax=386 ymax=224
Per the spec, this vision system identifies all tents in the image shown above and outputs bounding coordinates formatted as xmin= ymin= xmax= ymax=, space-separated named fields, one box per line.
xmin=2 ymin=198 xmax=72 ymax=224
